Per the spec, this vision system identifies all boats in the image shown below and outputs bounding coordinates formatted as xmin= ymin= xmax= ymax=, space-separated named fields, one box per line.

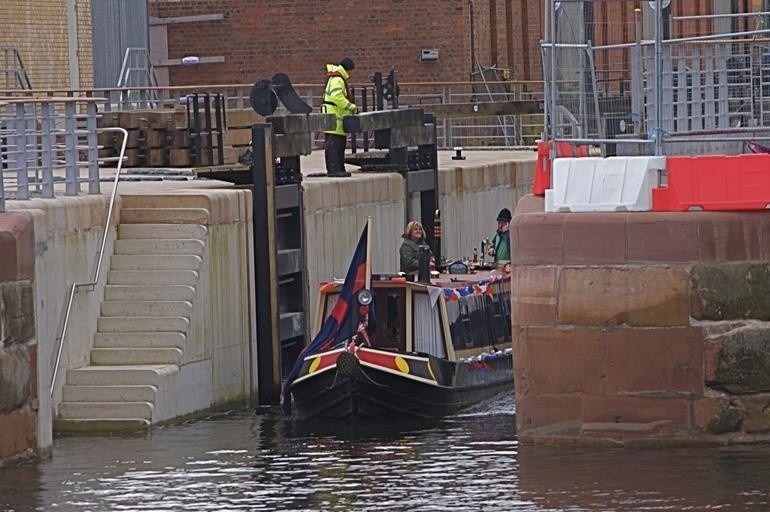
xmin=280 ymin=213 xmax=515 ymax=432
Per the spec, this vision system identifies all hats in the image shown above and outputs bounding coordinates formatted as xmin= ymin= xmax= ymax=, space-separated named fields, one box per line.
xmin=497 ymin=208 xmax=511 ymax=221
xmin=341 ymin=58 xmax=354 ymax=70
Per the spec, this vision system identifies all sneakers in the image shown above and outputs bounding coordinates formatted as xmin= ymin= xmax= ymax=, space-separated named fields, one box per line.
xmin=329 ymin=172 xmax=351 ymax=177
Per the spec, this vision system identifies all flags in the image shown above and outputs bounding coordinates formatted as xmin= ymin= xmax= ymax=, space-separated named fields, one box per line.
xmin=271 ymin=217 xmax=374 ymax=413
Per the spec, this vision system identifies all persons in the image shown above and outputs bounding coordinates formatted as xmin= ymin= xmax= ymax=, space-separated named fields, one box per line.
xmin=486 ymin=208 xmax=511 ymax=265
xmin=319 ymin=59 xmax=359 ymax=178
xmin=397 ymin=221 xmax=438 ymax=278
